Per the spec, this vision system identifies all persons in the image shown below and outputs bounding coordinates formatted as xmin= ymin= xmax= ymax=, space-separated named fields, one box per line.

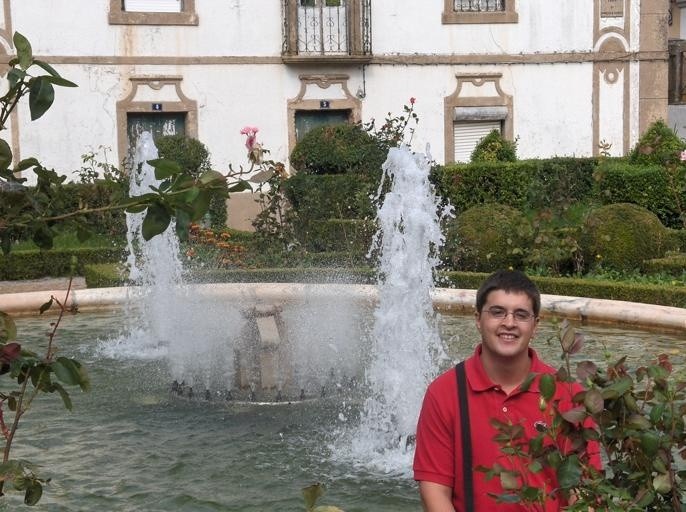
xmin=413 ymin=266 xmax=602 ymax=512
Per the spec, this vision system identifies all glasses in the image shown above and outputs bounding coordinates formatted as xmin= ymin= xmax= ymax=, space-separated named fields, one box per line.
xmin=481 ymin=309 xmax=537 ymax=322
